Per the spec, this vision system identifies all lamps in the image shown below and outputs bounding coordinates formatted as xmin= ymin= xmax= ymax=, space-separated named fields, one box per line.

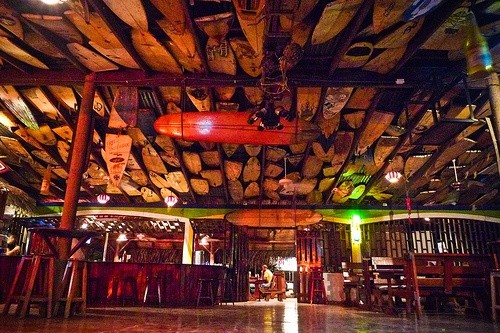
xmin=164 ymin=178 xmax=177 ymax=208
xmin=449 ymin=158 xmax=462 ymax=191
xmin=386 ymin=156 xmax=403 ymax=184
xmin=97 ymin=179 xmax=111 ymax=205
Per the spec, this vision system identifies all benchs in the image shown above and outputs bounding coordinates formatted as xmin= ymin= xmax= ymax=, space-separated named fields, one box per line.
xmin=342 ymin=261 xmax=369 ymax=306
xmin=403 ymin=252 xmax=499 ymax=320
xmin=368 ymin=256 xmax=410 ymax=309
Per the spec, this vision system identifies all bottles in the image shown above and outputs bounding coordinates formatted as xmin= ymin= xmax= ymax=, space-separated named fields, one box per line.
xmin=122 ymin=250 xmax=127 ymax=262
xmin=206 ymin=261 xmax=210 ymax=265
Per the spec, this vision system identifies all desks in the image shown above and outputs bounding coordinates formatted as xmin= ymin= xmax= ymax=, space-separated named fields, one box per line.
xmin=202 ymin=265 xmax=236 ymax=296
xmin=368 ymin=268 xmax=405 ymax=315
xmin=27 ymin=228 xmax=106 ymax=297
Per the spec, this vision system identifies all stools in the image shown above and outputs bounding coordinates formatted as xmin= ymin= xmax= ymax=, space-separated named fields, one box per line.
xmin=111 ymin=276 xmax=138 ymax=308
xmin=52 ymin=258 xmax=87 ymax=319
xmin=196 ymin=278 xmax=215 ymax=307
xmin=216 ymin=279 xmax=234 ymax=306
xmin=3 ymin=255 xmax=56 ymax=318
xmin=142 ymin=274 xmax=167 ymax=308
xmin=86 ymin=277 xmax=103 ymax=308
xmin=308 ymin=266 xmax=330 ymax=304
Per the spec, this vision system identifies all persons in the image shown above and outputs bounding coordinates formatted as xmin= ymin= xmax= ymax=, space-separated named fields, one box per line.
xmin=259 ymin=265 xmax=273 ymax=287
xmin=5 ymin=232 xmax=21 ymax=256
